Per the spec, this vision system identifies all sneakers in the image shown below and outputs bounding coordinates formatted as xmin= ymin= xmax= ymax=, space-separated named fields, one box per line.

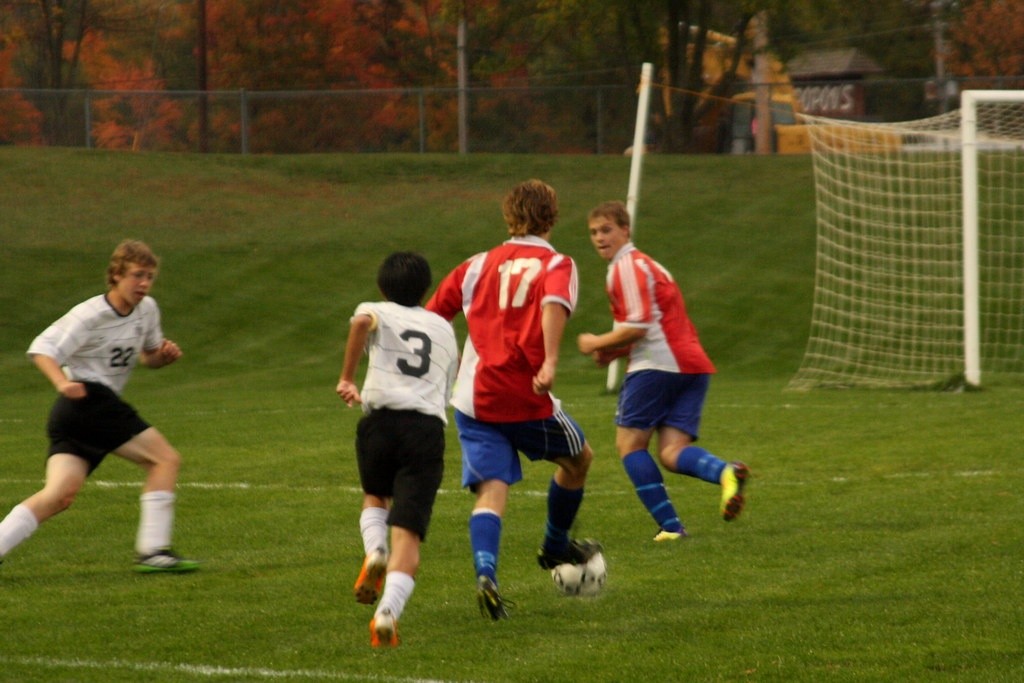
xmin=353 ymin=547 xmax=387 ymax=605
xmin=537 ymin=536 xmax=603 ymax=569
xmin=651 ymin=526 xmax=686 ymax=541
xmin=718 ymin=461 xmax=752 ymax=521
xmin=134 ymin=546 xmax=202 ymax=574
xmin=369 ymin=609 xmax=401 ymax=648
xmin=476 ymin=576 xmax=508 ymax=624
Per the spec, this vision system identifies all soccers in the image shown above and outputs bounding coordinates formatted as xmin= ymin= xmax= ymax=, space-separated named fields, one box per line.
xmin=551 ymin=552 xmax=606 ymax=594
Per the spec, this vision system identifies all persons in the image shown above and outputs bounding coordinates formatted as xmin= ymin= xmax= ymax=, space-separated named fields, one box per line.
xmin=423 ymin=178 xmax=607 ymax=622
xmin=335 ymin=250 xmax=462 ymax=646
xmin=575 ymin=199 xmax=748 ymax=542
xmin=0 ymin=240 xmax=208 ymax=576
